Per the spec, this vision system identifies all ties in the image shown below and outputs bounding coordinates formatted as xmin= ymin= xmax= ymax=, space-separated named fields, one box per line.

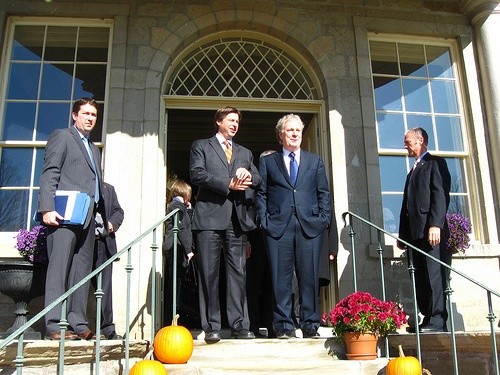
xmin=223 ymin=141 xmax=232 ymax=164
xmin=82 ymin=136 xmax=99 ymax=204
xmin=407 ymin=161 xmax=417 ymax=209
xmin=289 ymin=152 xmax=299 ymax=186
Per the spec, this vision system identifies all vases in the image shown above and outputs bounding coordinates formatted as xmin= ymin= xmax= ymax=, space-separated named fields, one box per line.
xmin=0 ymin=263 xmax=48 ymax=341
xmin=344 ymin=331 xmax=378 ymax=361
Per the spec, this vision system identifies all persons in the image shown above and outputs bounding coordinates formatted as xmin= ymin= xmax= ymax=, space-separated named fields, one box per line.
xmin=162 ymin=150 xmax=277 ymax=341
xmin=258 ymin=113 xmax=332 ymax=341
xmin=37 ymin=98 xmax=103 ymax=342
xmin=397 ymin=126 xmax=451 ymax=333
xmin=93 ymin=180 xmax=125 ymax=340
xmin=189 ymin=106 xmax=262 ymax=341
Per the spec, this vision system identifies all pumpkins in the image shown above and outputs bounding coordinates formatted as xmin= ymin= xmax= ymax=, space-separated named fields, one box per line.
xmin=153 ymin=314 xmax=194 ymax=364
xmin=386 ymin=344 xmax=421 ymax=375
xmin=129 ymin=347 xmax=168 ymax=375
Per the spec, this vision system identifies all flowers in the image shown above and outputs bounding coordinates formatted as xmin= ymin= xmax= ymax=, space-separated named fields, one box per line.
xmin=14 ymin=225 xmax=46 ymax=265
xmin=322 ymin=292 xmax=410 ymax=342
xmin=445 ymin=214 xmax=472 ymax=253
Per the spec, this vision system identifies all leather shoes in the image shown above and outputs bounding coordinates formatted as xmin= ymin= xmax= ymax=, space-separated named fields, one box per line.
xmin=406 ymin=323 xmax=425 ymax=333
xmin=277 ymin=329 xmax=292 ymax=338
xmin=303 ymin=329 xmax=320 ymax=338
xmin=78 ymin=330 xmax=106 ymax=340
xmin=105 ymin=332 xmax=122 ymax=340
xmin=204 ymin=330 xmax=221 ymax=341
xmin=231 ymin=329 xmax=255 ymax=338
xmin=44 ymin=330 xmax=81 ymax=340
xmin=419 ymin=324 xmax=448 ymax=333
